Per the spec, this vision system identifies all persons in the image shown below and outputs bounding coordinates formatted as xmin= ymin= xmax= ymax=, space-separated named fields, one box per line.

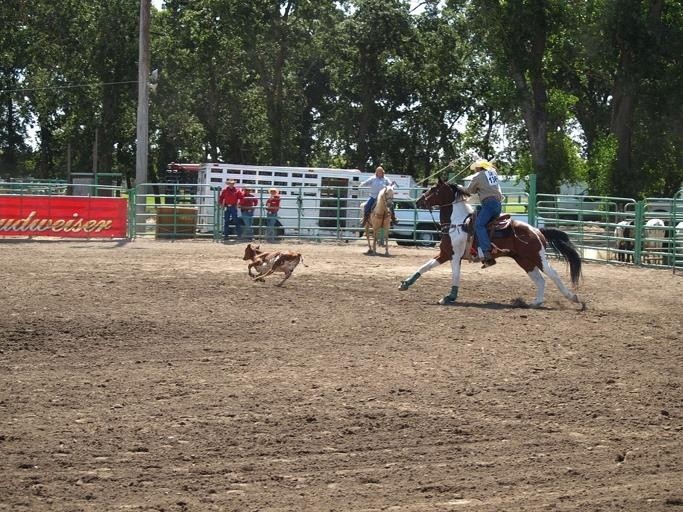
xmin=263 ymin=188 xmax=279 ymax=240
xmin=450 ymin=148 xmax=502 ymax=269
xmin=357 ymin=166 xmax=400 ymax=226
xmin=237 ymin=184 xmax=256 ymax=241
xmin=217 ymin=180 xmax=241 ymax=239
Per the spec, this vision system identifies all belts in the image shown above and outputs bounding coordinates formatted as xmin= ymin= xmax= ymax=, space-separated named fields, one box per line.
xmin=224 ymin=204 xmax=236 ymax=207
xmin=241 ymin=208 xmax=254 ymax=213
xmin=268 ymin=210 xmax=275 ymax=214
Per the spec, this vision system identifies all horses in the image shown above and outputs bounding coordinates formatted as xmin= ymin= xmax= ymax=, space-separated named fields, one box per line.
xmin=613 ymin=218 xmax=683 ymax=269
xmin=397 ymin=174 xmax=582 ymax=309
xmin=360 ymin=185 xmax=395 ymax=257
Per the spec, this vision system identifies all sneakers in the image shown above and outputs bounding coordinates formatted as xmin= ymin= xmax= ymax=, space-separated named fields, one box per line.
xmin=358 ymin=218 xmax=367 ymax=228
xmin=482 ymin=257 xmax=496 ymax=269
xmin=392 ymin=216 xmax=399 ymax=223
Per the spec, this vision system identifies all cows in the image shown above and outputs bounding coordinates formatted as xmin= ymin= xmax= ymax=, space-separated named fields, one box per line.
xmin=242 ymin=243 xmax=309 ymax=287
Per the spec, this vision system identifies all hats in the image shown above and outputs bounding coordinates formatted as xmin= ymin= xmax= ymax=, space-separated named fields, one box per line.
xmin=267 ymin=188 xmax=281 ymax=195
xmin=225 ymin=178 xmax=238 ymax=184
xmin=469 ymin=156 xmax=498 ymax=171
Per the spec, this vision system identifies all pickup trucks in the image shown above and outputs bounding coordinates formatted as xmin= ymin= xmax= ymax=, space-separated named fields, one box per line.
xmin=392 ymin=200 xmax=546 ymax=247
